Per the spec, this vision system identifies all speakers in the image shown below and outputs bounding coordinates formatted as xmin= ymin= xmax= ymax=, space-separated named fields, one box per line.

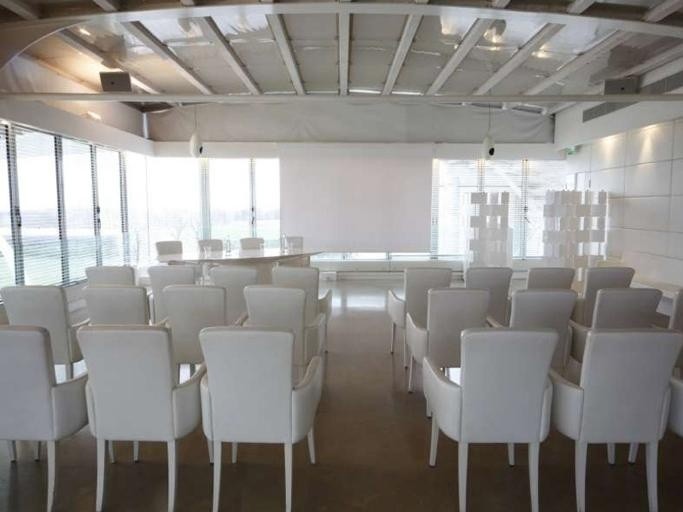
xmin=98 ymin=71 xmax=131 ymax=93
xmin=605 ymin=77 xmax=637 ymax=95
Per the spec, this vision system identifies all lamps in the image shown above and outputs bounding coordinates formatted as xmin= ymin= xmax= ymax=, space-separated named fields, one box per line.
xmin=185 ymin=102 xmax=203 ymax=158
xmin=483 ymin=91 xmax=496 ymax=163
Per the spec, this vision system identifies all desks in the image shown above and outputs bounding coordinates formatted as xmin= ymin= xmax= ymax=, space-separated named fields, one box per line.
xmin=157 ymin=247 xmax=325 ymax=267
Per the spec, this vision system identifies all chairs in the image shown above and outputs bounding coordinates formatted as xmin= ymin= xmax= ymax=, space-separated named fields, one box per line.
xmin=0 ymin=327 xmax=88 ymax=511
xmin=548 ymin=328 xmax=681 ymax=512
xmin=193 ymin=328 xmax=324 ymax=511
xmin=386 ymin=233 xmax=681 ymax=419
xmin=0 ymin=234 xmax=333 ymax=419
xmin=73 ymin=327 xmax=206 ymax=512
xmin=420 ymin=328 xmax=559 ymax=512
